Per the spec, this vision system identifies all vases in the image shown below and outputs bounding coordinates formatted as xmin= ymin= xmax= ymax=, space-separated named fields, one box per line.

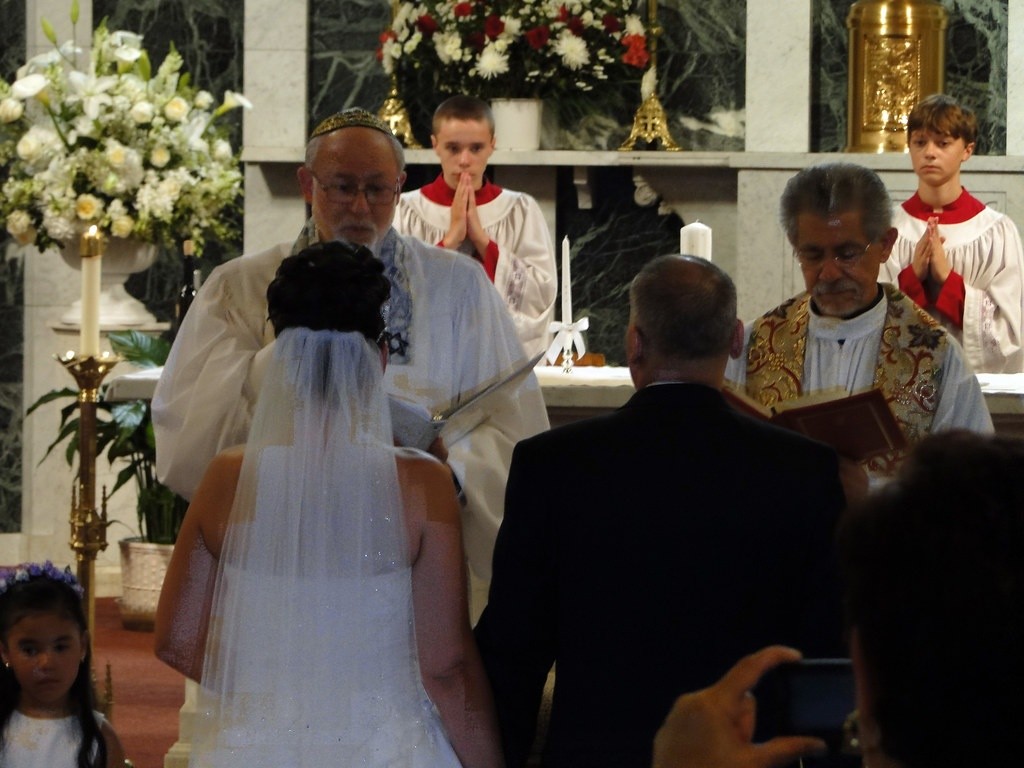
xmin=488 ymin=96 xmax=544 ymax=151
xmin=57 ymin=241 xmax=160 ymax=327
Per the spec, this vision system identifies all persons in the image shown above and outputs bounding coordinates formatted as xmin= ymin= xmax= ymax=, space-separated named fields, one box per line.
xmin=0 ymin=560 xmax=127 ymax=768
xmin=720 ymin=159 xmax=995 ymax=514
xmin=474 ymin=253 xmax=848 ymax=768
xmin=154 ymin=238 xmax=504 ymax=768
xmin=149 ymin=105 xmax=552 ymax=634
xmin=876 ymin=93 xmax=1024 ymax=375
xmin=389 ymin=93 xmax=558 ymax=365
xmin=652 ymin=425 xmax=1024 ymax=768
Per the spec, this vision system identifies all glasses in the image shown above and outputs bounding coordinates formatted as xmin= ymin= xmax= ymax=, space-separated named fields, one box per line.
xmin=308 ymin=171 xmax=401 ymax=205
xmin=791 ymin=235 xmax=875 ymax=266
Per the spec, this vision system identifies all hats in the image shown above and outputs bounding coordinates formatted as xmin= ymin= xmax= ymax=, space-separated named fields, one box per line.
xmin=310 ymin=107 xmax=392 ymax=137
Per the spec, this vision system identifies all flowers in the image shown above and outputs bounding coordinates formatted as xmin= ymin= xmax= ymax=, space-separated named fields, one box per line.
xmin=0 ymin=1 xmax=254 ymax=263
xmin=0 ymin=559 xmax=85 ymax=599
xmin=377 ymin=0 xmax=661 ymax=125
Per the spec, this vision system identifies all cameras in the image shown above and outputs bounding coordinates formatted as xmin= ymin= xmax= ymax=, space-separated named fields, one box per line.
xmin=781 ymin=659 xmax=858 ymax=736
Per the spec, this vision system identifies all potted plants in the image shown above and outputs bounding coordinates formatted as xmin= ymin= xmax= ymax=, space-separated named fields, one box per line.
xmin=25 ymin=333 xmax=189 ymax=633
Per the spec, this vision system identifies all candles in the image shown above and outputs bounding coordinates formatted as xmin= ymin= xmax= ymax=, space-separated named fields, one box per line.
xmin=79 ymin=224 xmax=101 ymax=357
xmin=680 ymin=219 xmax=712 ymax=261
xmin=561 ymin=234 xmax=572 ymax=325
